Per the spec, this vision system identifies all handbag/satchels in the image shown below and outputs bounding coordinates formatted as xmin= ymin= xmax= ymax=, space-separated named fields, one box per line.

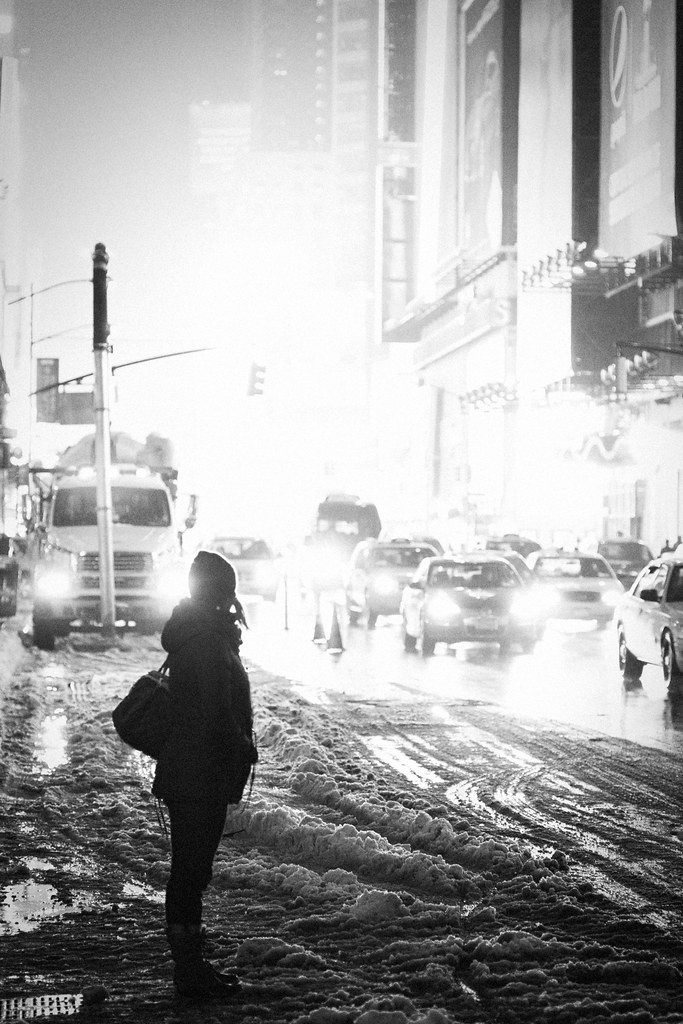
xmin=111 ymin=628 xmax=240 ymax=761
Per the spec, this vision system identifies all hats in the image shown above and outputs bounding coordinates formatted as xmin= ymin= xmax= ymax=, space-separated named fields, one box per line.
xmin=188 ymin=551 xmax=236 ymax=600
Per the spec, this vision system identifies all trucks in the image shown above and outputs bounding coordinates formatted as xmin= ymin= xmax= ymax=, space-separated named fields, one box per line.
xmin=30 ymin=465 xmax=202 ymax=653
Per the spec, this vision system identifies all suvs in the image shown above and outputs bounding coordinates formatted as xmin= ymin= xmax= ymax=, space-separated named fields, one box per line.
xmin=347 ymin=537 xmax=441 ymax=628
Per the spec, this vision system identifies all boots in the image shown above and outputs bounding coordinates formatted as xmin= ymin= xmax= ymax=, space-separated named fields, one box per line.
xmin=166 ymin=926 xmax=243 ymax=998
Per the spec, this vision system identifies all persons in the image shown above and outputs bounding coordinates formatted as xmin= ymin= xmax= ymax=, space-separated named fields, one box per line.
xmin=152 ymin=548 xmax=261 ymax=1003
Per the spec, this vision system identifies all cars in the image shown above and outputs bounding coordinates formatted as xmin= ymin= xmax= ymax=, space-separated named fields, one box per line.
xmin=525 ymin=551 xmax=623 ymax=630
xmin=600 ymin=539 xmax=656 ymax=590
xmin=400 ymin=556 xmax=531 ymax=658
xmin=489 ymin=536 xmax=544 ymax=566
xmin=477 ymin=552 xmax=547 ymax=640
xmin=613 ymin=558 xmax=683 ymax=689
xmin=216 ymin=539 xmax=282 ymax=603
